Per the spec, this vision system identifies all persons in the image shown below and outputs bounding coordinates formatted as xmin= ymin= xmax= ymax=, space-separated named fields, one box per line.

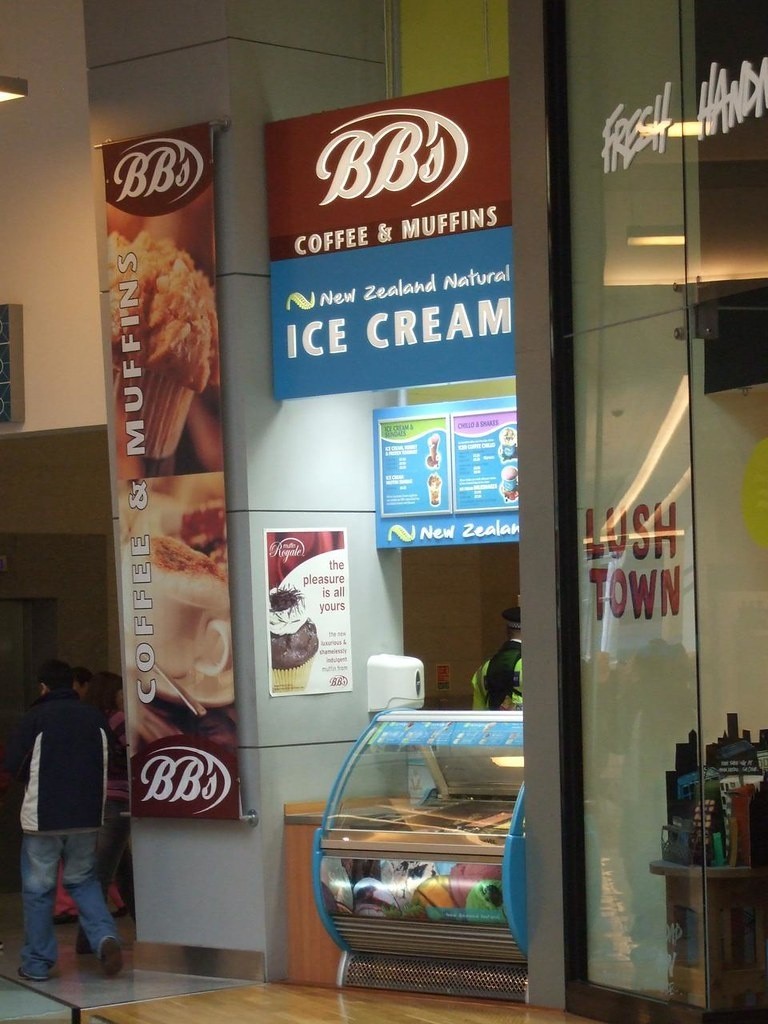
xmin=471 ymin=607 xmax=521 ymax=710
xmin=4 ymin=657 xmax=127 ymax=982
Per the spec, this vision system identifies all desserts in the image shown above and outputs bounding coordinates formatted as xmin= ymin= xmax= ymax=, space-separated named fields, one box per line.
xmin=268 ymin=585 xmax=319 ymax=691
xmin=105 ymin=230 xmax=219 ymax=458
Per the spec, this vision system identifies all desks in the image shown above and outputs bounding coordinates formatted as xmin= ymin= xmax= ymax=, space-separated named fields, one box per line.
xmin=650 ymin=859 xmax=768 ymax=1011
xmin=284 ymin=796 xmax=413 ymax=983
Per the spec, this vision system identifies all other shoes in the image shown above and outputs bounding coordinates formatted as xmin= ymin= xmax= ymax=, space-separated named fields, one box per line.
xmin=111 ymin=906 xmax=127 ymax=918
xmin=53 ymin=914 xmax=78 ymax=925
xmin=18 ymin=967 xmax=49 ymax=982
xmin=102 ymin=940 xmax=123 ymax=973
xmin=76 ymin=943 xmax=94 ymax=954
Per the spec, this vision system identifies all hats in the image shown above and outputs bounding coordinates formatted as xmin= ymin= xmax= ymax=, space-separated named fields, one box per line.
xmin=502 ymin=606 xmax=521 ymax=630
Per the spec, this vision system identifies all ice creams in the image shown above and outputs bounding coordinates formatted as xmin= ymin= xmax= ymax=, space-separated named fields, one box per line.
xmin=499 ymin=427 xmax=518 ymax=457
xmin=428 ymin=434 xmax=439 ymax=462
xmin=501 ymin=466 xmax=518 ymax=491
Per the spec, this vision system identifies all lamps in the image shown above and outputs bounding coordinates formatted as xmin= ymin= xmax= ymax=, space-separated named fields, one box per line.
xmin=626 ymin=225 xmax=685 ymax=246
xmin=1 ymin=76 xmax=28 ymax=102
